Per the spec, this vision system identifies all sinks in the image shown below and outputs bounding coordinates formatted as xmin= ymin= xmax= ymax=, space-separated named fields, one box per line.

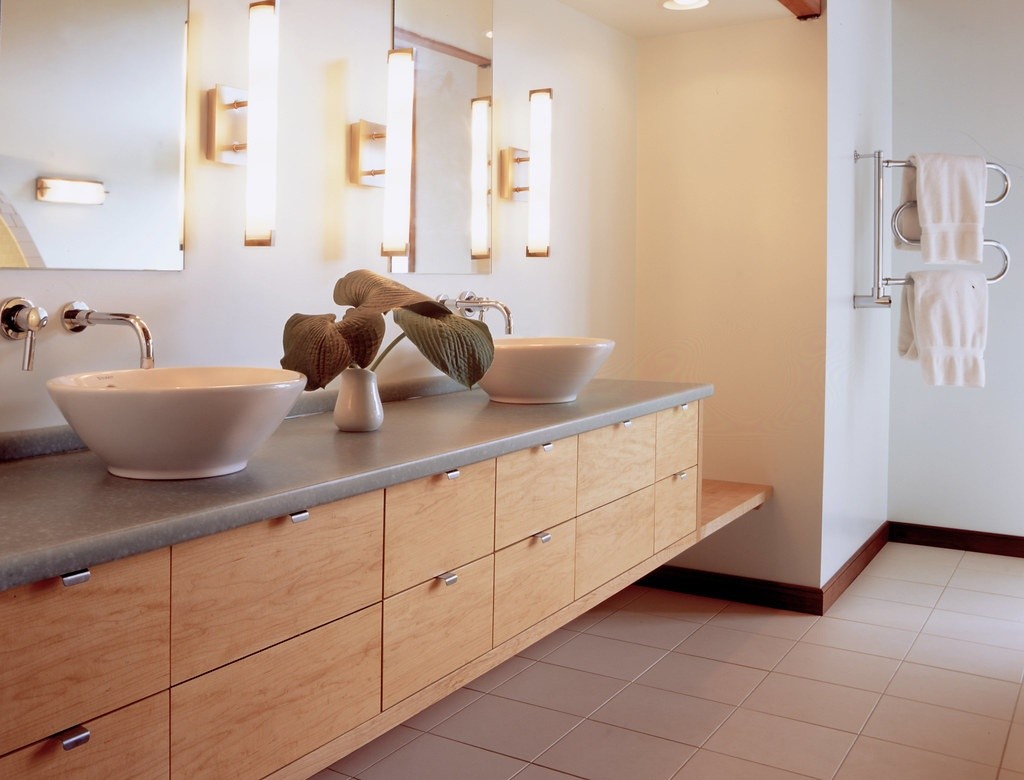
xmin=474 ymin=335 xmax=617 ymax=405
xmin=44 ymin=365 xmax=307 ymax=484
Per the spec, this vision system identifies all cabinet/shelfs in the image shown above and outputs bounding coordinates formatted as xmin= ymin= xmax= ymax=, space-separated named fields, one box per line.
xmin=1 ymin=399 xmax=774 ymax=779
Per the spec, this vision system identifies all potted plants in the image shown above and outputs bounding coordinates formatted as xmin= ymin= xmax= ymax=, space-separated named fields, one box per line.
xmin=279 ymin=269 xmax=495 ymax=432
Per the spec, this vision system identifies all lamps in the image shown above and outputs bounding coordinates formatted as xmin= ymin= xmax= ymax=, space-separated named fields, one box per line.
xmin=350 ymin=45 xmax=415 ymax=257
xmin=210 ymin=3 xmax=280 ymax=247
xmin=466 ymin=95 xmax=493 ymax=260
xmin=500 ymin=88 xmax=552 ymax=257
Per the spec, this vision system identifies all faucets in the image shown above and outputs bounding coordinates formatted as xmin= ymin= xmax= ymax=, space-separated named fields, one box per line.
xmin=59 ymin=298 xmax=156 ymax=370
xmin=435 ymin=294 xmax=514 ymax=336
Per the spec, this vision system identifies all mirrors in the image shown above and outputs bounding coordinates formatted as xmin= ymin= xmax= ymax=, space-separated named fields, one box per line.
xmin=389 ymin=0 xmax=493 ymax=274
xmin=0 ymin=0 xmax=189 ymax=271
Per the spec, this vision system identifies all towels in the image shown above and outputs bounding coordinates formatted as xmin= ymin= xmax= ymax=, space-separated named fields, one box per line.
xmin=894 ymin=151 xmax=988 ymax=266
xmin=896 ymin=268 xmax=990 ymax=390
